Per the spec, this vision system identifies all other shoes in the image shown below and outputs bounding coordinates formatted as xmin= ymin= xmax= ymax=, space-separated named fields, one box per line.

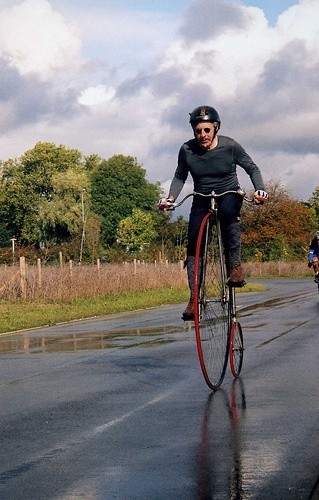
xmin=181 ymin=294 xmax=198 ymax=316
xmin=315 ymin=277 xmax=319 ymax=283
xmin=228 ymin=262 xmax=244 ymax=283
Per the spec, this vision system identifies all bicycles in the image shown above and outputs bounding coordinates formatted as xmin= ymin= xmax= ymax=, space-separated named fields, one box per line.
xmin=166 ymin=189 xmax=266 ymax=389
xmin=311 ymin=261 xmax=319 ymax=290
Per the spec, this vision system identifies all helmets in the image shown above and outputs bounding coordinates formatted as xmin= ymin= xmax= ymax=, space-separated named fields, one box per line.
xmin=189 ymin=105 xmax=221 ymax=124
xmin=314 ymin=231 xmax=319 ymax=240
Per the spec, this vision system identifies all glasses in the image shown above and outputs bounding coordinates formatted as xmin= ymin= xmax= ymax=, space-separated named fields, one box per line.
xmin=194 ymin=127 xmax=211 ymax=135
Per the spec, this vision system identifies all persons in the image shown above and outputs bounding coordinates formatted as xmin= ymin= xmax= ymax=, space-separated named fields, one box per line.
xmin=158 ymin=106 xmax=271 ymax=322
xmin=307 ymin=231 xmax=319 ymax=282
